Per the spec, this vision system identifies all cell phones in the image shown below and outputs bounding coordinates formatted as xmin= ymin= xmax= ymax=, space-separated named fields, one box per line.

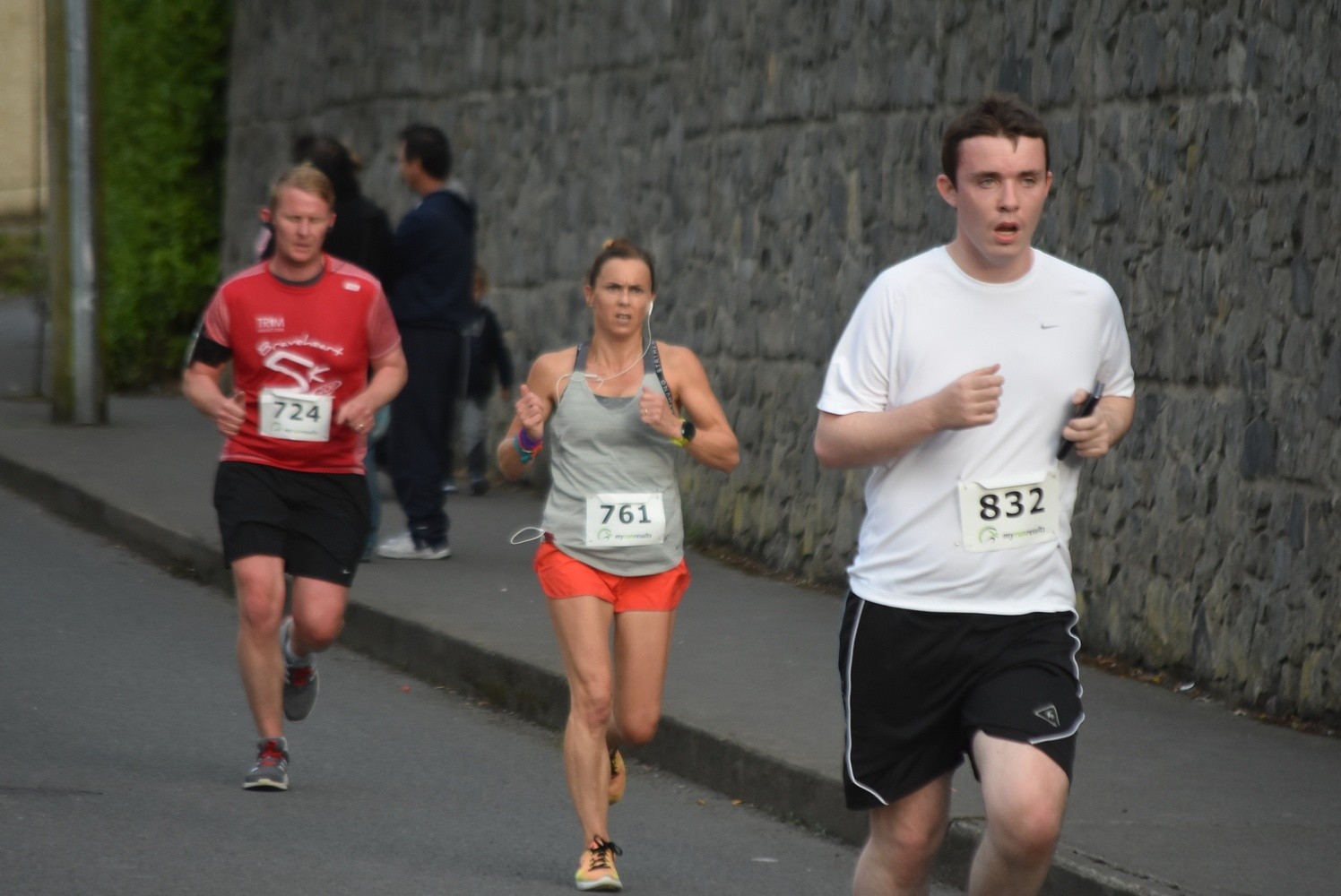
xmin=1057 ymin=380 xmax=1104 ymax=460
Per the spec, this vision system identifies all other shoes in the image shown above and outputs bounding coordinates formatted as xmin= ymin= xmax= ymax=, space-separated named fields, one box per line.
xmin=374 ymin=531 xmax=452 ymax=559
xmin=470 ymin=476 xmax=490 ymax=496
xmin=443 ymin=480 xmax=458 ymax=492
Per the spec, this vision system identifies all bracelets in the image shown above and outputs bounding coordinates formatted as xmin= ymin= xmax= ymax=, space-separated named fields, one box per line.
xmin=512 ymin=425 xmax=543 ymax=462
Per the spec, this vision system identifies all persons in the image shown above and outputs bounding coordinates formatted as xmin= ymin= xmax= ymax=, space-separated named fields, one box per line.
xmin=178 ymin=166 xmax=409 ymax=793
xmin=811 ymin=99 xmax=1136 ymax=895
xmin=255 ymin=119 xmax=482 ymax=559
xmin=495 ymin=240 xmax=741 ymax=895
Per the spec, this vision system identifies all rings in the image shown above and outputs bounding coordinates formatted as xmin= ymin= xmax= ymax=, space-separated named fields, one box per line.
xmin=359 ymin=423 xmax=365 ymax=429
xmin=644 ymin=409 xmax=649 ymax=416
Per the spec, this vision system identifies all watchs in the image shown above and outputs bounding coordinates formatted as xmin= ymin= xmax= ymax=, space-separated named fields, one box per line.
xmin=668 ymin=419 xmax=696 ymax=447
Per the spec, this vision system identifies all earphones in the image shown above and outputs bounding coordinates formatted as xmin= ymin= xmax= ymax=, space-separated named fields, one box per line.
xmin=648 ymin=301 xmax=654 ymax=315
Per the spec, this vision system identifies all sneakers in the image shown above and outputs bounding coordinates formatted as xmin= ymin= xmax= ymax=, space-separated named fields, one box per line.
xmin=607 ymin=750 xmax=625 ymax=802
xmin=277 ymin=617 xmax=319 ymax=721
xmin=242 ymin=734 xmax=289 ymax=790
xmin=574 ymin=833 xmax=623 ymax=889
